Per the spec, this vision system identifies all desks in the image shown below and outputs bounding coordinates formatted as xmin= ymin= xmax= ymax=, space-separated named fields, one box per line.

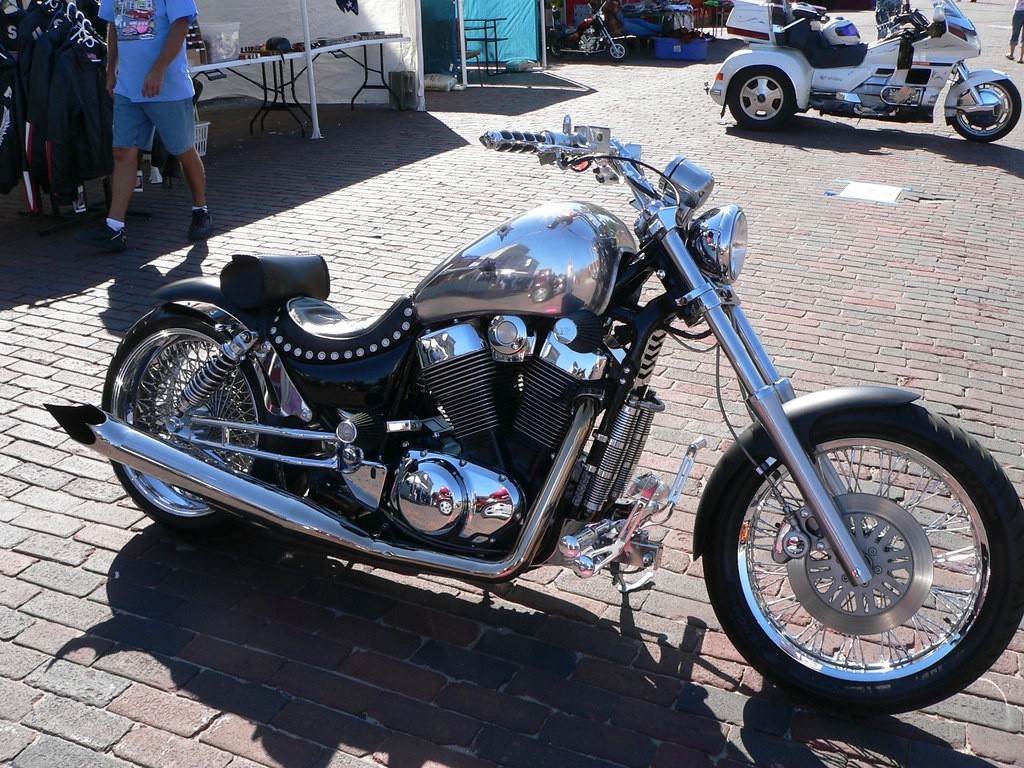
xmin=189 ymin=50 xmax=307 ymax=140
xmin=260 ymin=37 xmax=411 ymax=140
xmin=624 ymin=9 xmax=698 ymax=37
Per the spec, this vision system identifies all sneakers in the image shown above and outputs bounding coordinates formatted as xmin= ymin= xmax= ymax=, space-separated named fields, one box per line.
xmin=74 ymin=220 xmax=129 ymax=251
xmin=188 ymin=208 xmax=214 ymax=240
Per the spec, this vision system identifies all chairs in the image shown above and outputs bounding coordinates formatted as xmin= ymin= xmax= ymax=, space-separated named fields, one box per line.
xmin=434 ymin=18 xmax=485 ymax=88
xmin=598 ymin=4 xmax=641 ymax=54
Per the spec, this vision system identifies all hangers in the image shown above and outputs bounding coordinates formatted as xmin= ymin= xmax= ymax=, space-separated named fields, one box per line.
xmin=45 ymin=0 xmax=99 ymax=47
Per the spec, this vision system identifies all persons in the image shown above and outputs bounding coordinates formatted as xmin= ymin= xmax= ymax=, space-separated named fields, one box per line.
xmin=1005 ymin=0 xmax=1024 ymax=63
xmin=875 ymin=0 xmax=903 ymax=40
xmin=74 ymin=0 xmax=213 ymax=253
xmin=603 ymin=0 xmax=674 ymax=35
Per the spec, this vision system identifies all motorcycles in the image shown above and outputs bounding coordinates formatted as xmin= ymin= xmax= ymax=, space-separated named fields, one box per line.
xmin=547 ymin=0 xmax=629 ymax=63
xmin=704 ymin=0 xmax=1021 ymax=142
xmin=43 ymin=115 xmax=1024 ymax=719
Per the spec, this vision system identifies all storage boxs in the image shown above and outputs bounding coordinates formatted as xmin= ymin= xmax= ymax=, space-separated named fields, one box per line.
xmin=187 ymin=48 xmax=201 ymax=67
xmin=318 ymin=30 xmax=403 ymax=46
xmin=192 ymin=118 xmax=211 ymax=158
xmin=653 ymin=37 xmax=713 ymax=63
xmin=199 ymin=20 xmax=241 ymax=63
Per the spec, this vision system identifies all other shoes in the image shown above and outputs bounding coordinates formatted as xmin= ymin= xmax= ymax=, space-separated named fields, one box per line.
xmin=1017 ymin=59 xmax=1024 ymax=63
xmin=1005 ymin=53 xmax=1014 ymax=60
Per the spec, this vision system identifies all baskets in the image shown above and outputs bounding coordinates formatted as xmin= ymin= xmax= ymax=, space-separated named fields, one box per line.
xmin=194 ymin=120 xmax=211 ymax=156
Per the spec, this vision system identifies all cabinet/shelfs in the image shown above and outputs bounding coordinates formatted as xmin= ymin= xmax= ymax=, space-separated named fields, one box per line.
xmin=464 ymin=18 xmax=509 ymax=75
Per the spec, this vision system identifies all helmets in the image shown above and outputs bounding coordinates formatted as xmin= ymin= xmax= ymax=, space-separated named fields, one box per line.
xmin=821 ymin=17 xmax=861 ymax=46
xmin=266 ymin=36 xmax=293 ymax=53
